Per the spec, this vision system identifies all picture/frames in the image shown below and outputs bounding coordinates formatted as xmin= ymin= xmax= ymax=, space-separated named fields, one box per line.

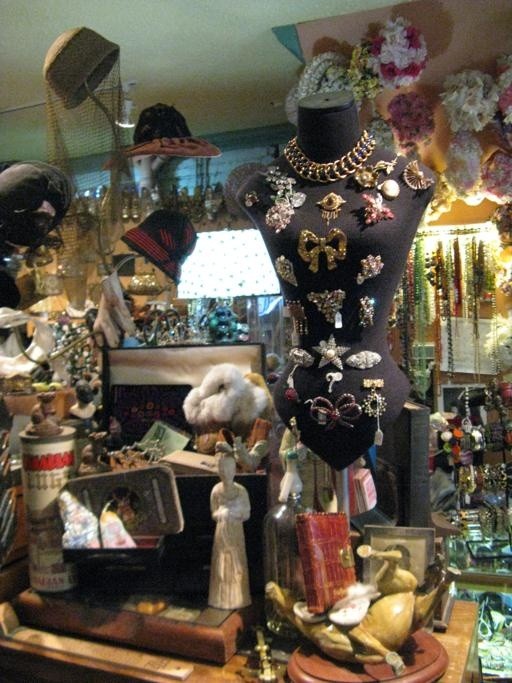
xmin=363 ymin=526 xmax=434 ymax=597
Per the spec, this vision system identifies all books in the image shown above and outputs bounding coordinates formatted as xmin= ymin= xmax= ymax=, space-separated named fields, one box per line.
xmin=295 ymin=510 xmax=356 ymax=614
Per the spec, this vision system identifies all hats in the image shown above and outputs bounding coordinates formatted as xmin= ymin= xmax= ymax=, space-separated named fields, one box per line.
xmin=0 ymin=160 xmax=73 ymax=247
xmin=120 ymin=209 xmax=197 ymax=283
xmin=42 ymin=26 xmax=120 ymax=110
xmin=99 ymin=102 xmax=223 ymax=171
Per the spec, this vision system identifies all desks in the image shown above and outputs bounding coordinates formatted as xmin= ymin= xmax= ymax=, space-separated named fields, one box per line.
xmin=0 ymin=600 xmax=479 ymax=683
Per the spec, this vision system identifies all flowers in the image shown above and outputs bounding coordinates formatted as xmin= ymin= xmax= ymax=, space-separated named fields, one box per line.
xmin=388 ymin=93 xmax=436 ymax=153
xmin=491 ymin=54 xmax=512 ymax=151
xmin=441 ymin=69 xmax=500 ymax=133
xmin=441 ymin=131 xmax=482 ymax=199
xmin=491 ymin=207 xmax=512 ymax=234
xmin=324 ymin=47 xmax=383 ymax=102
xmin=481 ymin=151 xmax=512 ymax=206
xmin=421 ymin=170 xmax=457 ymax=223
xmin=367 ymin=119 xmax=395 ymax=154
xmin=367 ymin=16 xmax=428 ymax=90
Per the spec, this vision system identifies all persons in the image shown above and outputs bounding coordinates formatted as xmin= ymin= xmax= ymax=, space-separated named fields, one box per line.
xmin=236 ymin=89 xmax=438 ymax=473
xmin=207 ymin=441 xmax=253 ymax=610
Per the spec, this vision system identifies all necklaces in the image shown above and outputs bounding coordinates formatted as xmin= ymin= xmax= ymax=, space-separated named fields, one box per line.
xmin=283 ymin=130 xmax=377 ymax=183
xmin=389 ymin=236 xmax=501 ymax=385
